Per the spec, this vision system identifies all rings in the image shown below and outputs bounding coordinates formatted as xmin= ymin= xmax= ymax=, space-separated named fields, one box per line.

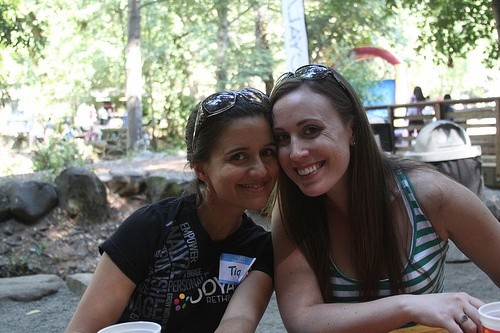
xmin=459 ymin=314 xmax=469 ymax=325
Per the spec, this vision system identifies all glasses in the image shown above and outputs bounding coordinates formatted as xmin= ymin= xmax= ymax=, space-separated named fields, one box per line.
xmin=269 ymin=64 xmax=349 ymax=96
xmin=191 ymin=87 xmax=273 ymax=152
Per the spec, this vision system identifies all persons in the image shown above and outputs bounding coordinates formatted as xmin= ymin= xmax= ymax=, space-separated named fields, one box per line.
xmin=440 ymin=93 xmax=455 ymax=120
xmin=404 ymin=85 xmax=427 ymax=137
xmin=44 ymin=101 xmax=114 ymax=141
xmin=65 ymin=88 xmax=281 ymax=333
xmin=268 ymin=65 xmax=500 ymax=333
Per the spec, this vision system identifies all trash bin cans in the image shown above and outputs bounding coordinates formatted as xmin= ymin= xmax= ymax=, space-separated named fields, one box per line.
xmin=403 ymin=119 xmax=486 ymax=263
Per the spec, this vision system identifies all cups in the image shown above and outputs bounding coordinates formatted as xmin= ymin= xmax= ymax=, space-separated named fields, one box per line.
xmin=97 ymin=321 xmax=162 ymax=333
xmin=478 ymin=301 xmax=500 ymax=333
